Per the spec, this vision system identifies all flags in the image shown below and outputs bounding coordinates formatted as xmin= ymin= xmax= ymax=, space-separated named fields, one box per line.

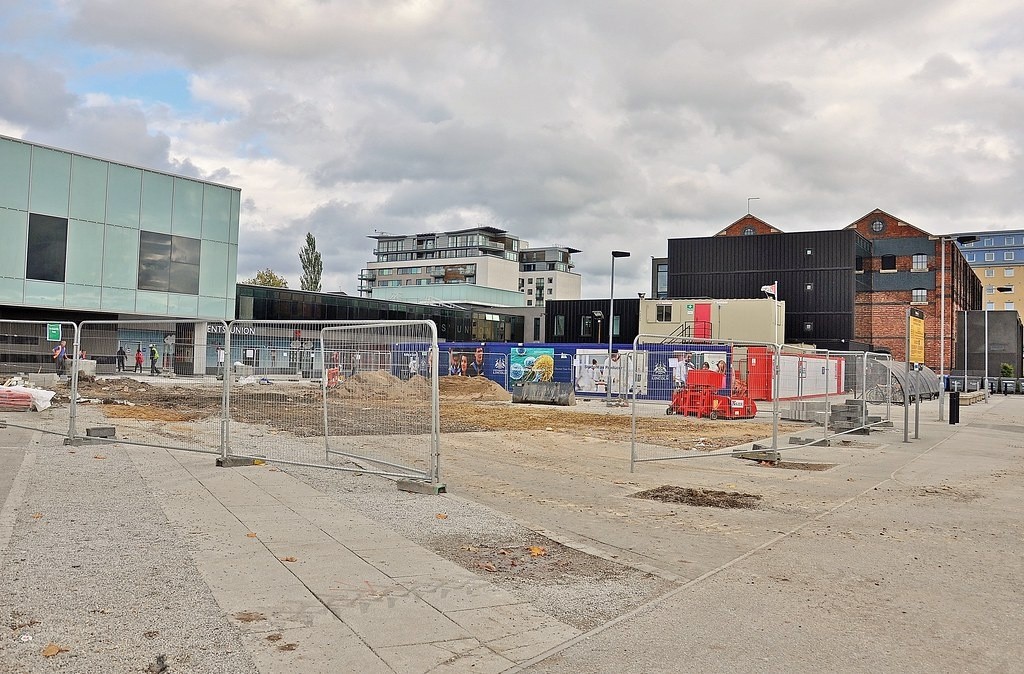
xmin=761 ymin=284 xmax=775 ymax=294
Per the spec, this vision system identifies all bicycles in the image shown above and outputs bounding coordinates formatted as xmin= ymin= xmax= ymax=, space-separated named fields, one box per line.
xmin=862 ymin=382 xmax=911 ymax=407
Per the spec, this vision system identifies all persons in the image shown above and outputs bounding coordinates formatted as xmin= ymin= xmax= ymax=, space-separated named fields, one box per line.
xmin=117 ymin=347 xmax=128 ymax=372
xmin=673 ymin=353 xmax=694 ymax=385
xmin=133 ymin=349 xmax=144 ymax=373
xmin=409 ymin=356 xmax=416 ymax=378
xmin=589 ymin=360 xmax=601 ymax=381
xmin=603 ymin=349 xmax=634 ymax=392
xmin=457 ymin=354 xmax=470 ymax=378
xmin=149 ymin=344 xmax=161 ymax=376
xmin=52 ymin=341 xmax=69 ymax=375
xmin=79 ymin=350 xmax=87 ymax=360
xmin=351 ymin=353 xmax=359 ymax=376
xmin=450 ymin=353 xmax=462 ymax=376
xmin=717 ymin=361 xmax=727 ymax=375
xmin=702 ymin=362 xmax=710 ymax=370
xmin=469 ymin=347 xmax=485 ymax=377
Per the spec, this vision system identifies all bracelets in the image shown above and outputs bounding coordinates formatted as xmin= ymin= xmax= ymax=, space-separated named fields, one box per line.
xmin=66 ymin=357 xmax=68 ymax=359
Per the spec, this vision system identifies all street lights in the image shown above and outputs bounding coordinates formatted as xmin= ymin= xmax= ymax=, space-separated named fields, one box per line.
xmin=937 ymin=234 xmax=981 ymax=422
xmin=605 ymin=250 xmax=631 ymax=405
xmin=983 ymin=285 xmax=1013 ymax=403
